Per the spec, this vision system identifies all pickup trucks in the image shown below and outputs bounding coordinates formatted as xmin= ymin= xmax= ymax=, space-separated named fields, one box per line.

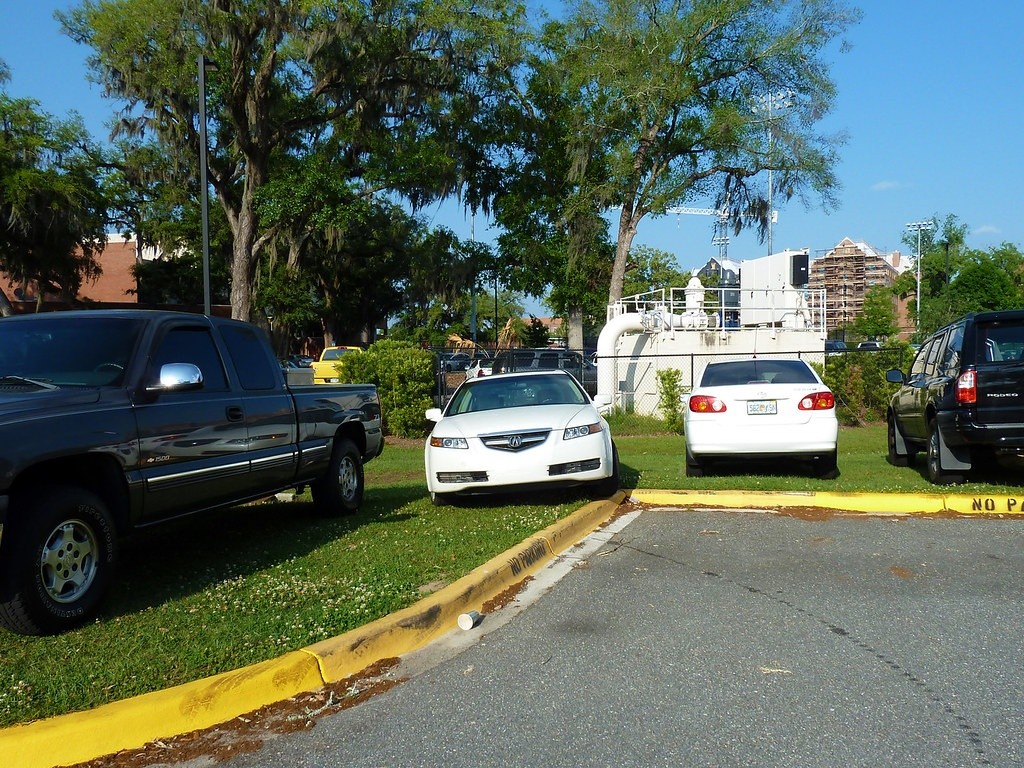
xmin=310 ymin=346 xmax=366 ymax=384
xmin=0 ymin=310 xmax=383 ymax=639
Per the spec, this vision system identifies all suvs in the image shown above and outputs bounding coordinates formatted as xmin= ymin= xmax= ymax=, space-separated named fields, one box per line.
xmin=887 ymin=309 xmax=1024 ymax=486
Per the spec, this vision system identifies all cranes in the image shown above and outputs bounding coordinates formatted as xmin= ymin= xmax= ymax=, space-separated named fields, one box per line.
xmin=608 ymin=193 xmax=778 ymax=262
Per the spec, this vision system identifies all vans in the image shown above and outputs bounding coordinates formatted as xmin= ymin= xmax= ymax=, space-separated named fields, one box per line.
xmin=825 ymin=340 xmax=847 ymax=353
xmin=492 ymin=350 xmax=597 ymax=395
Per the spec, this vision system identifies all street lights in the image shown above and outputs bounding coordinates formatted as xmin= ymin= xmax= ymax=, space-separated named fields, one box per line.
xmin=906 ymin=221 xmax=933 ymax=340
xmin=751 ymin=88 xmax=796 ymax=256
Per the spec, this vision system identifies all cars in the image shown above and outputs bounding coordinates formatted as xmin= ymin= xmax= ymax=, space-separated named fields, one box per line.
xmin=857 ymin=342 xmax=880 ymax=353
xmin=277 ymin=361 xmax=298 ymax=370
xmin=588 ymin=351 xmax=597 ymax=367
xmin=910 ymin=344 xmax=922 ymax=349
xmin=288 ymin=354 xmax=314 ymax=367
xmin=465 ymin=359 xmax=506 ymax=380
xmin=442 ymin=353 xmax=470 ymax=373
xmin=425 ymin=368 xmax=620 ymax=506
xmin=678 ymin=357 xmax=838 ymax=479
